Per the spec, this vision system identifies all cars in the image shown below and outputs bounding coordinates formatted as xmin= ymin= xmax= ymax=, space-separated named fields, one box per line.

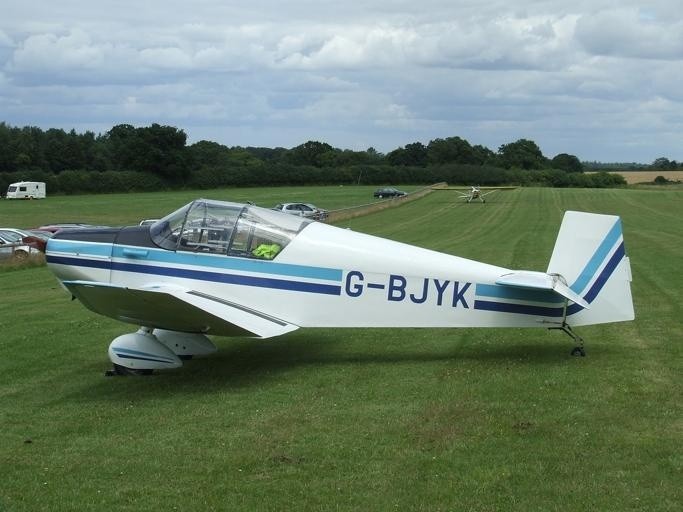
xmin=265 ymin=203 xmax=327 ymax=222
xmin=373 ymin=187 xmax=407 ymax=199
xmin=0 ymin=223 xmax=109 ymax=261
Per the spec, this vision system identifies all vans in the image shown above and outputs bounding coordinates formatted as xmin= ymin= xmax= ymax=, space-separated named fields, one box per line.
xmin=140 ymin=219 xmax=160 ymax=227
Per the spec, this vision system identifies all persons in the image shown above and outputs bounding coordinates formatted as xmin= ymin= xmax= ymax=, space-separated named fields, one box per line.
xmin=208 ymin=218 xmax=221 ymax=250
xmin=221 ymin=216 xmax=235 ymax=250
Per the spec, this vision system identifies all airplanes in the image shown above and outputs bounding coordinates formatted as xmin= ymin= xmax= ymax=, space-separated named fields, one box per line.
xmin=22 ymin=199 xmax=637 ymax=377
xmin=430 ymin=186 xmax=517 ymax=204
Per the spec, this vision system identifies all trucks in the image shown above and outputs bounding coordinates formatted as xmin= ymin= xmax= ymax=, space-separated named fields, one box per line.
xmin=6 ymin=181 xmax=46 ymax=201
